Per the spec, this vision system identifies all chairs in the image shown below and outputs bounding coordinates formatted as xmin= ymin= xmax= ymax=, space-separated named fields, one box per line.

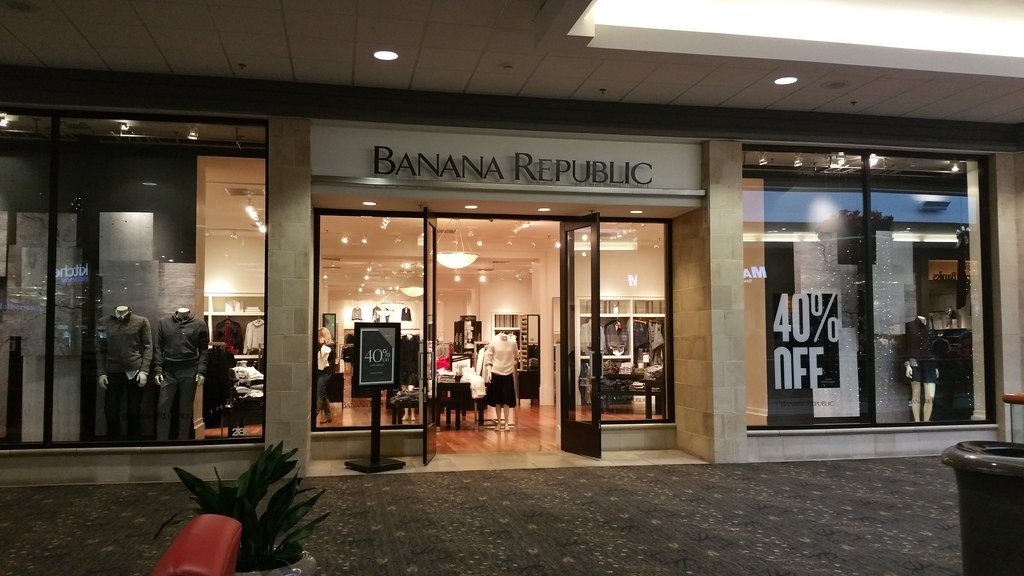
xmin=151 ymin=515 xmax=242 ymax=576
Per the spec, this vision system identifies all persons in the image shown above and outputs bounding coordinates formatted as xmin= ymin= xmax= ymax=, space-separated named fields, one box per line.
xmin=485 ymin=333 xmax=518 ymax=431
xmin=316 ymin=328 xmax=335 ymax=422
xmin=95 ymin=306 xmax=153 ymax=438
xmin=154 ymin=308 xmax=209 ymax=440
xmin=903 ymin=316 xmax=939 ymax=422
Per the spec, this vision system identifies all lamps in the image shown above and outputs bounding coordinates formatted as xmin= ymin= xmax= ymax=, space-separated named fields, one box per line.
xmin=792 ymin=152 xmax=803 ymax=166
xmin=573 ymin=227 xmax=638 ymax=251
xmin=950 ymin=159 xmax=960 ymax=172
xmin=758 ymin=152 xmax=768 ymax=166
xmin=187 ymin=126 xmax=199 ymax=141
xmin=398 ymin=262 xmax=423 ymax=297
xmin=430 ymin=219 xmax=481 ymax=270
xmin=830 ymin=151 xmax=846 ymax=169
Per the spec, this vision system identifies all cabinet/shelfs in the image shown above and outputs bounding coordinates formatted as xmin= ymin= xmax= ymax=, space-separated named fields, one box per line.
xmin=203 ymin=293 xmax=265 ymax=358
xmin=575 ymin=296 xmax=666 ymax=406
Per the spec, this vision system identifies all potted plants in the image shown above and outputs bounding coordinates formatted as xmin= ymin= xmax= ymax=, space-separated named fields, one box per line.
xmin=152 ymin=440 xmax=332 ymax=576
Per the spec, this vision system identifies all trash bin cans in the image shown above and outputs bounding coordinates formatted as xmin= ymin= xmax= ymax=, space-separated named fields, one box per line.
xmin=944 ymin=441 xmax=1024 ymax=576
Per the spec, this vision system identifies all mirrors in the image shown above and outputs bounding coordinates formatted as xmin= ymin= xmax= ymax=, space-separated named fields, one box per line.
xmin=323 ymin=313 xmax=337 ymax=350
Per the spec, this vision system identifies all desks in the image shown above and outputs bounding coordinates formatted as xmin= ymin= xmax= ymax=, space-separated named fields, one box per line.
xmin=639 ymin=379 xmax=667 ymax=419
xmin=579 ymin=374 xmax=662 ymax=414
xmin=391 ymin=375 xmax=489 ymax=430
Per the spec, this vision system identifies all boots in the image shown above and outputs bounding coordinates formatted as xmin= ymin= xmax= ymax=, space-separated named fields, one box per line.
xmin=321 ymin=398 xmax=332 ymax=423
xmin=316 ymin=401 xmax=323 ymax=416
